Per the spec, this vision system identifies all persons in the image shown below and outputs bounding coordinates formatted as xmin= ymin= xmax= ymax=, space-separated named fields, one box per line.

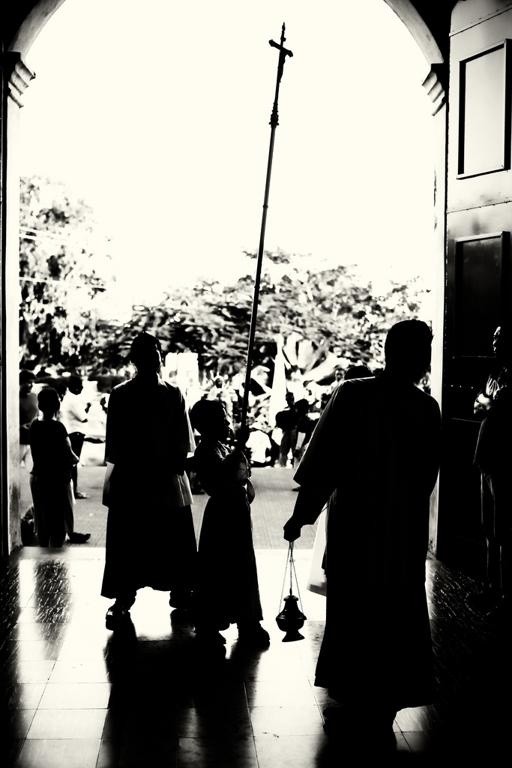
xmin=98 ymin=376 xmax=124 ymax=415
xmin=246 ymin=420 xmax=272 ymax=466
xmin=275 ymin=411 xmax=322 ymax=450
xmin=101 ymin=332 xmax=197 ymax=631
xmin=189 ymin=399 xmax=270 ymax=642
xmin=282 ymin=320 xmax=446 ymax=730
xmin=345 ymin=365 xmax=374 ymax=380
xmin=466 ymin=322 xmax=512 ymax=619
xmin=28 ymin=387 xmax=91 ymax=548
xmin=58 ymin=373 xmax=91 ymax=499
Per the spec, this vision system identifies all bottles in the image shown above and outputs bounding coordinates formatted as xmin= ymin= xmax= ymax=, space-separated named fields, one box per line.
xmin=276 ymin=587 xmax=310 ymax=645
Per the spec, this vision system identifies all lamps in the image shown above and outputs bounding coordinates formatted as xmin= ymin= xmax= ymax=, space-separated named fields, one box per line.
xmin=275 ymin=540 xmax=306 ymax=642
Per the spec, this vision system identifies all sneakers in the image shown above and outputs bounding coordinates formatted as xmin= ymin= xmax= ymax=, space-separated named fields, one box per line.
xmin=106 ymin=596 xmax=273 ymax=646
